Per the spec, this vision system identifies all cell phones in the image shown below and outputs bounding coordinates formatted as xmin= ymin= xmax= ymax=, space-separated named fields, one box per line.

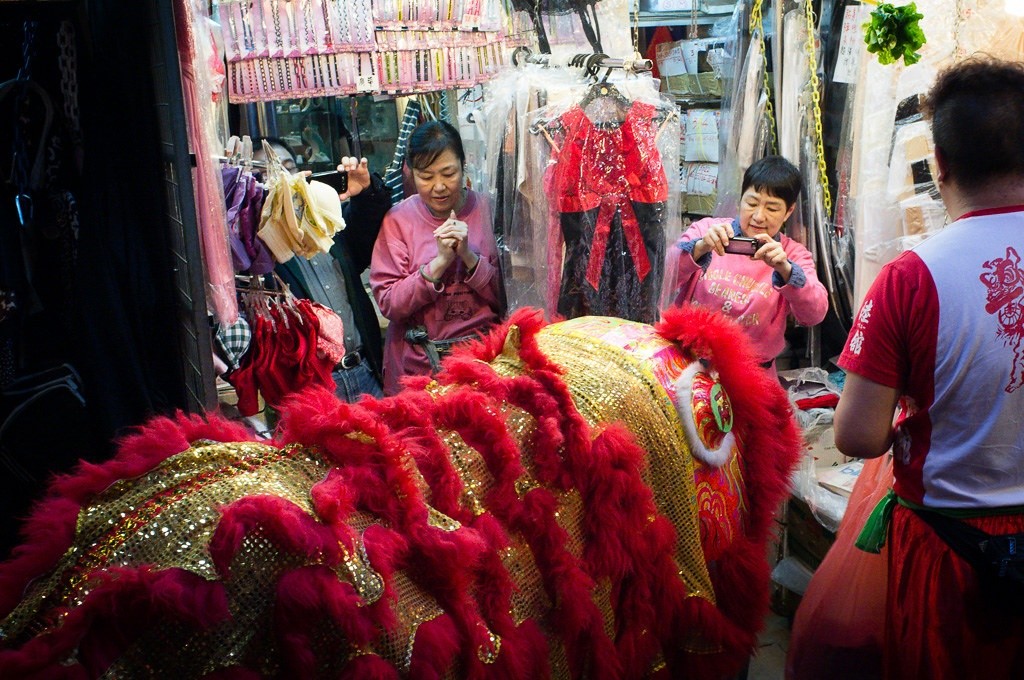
xmin=724 ymin=238 xmax=759 ymax=256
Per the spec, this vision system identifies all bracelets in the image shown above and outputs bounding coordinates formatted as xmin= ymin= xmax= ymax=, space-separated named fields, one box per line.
xmin=420 ymin=264 xmax=442 ymax=283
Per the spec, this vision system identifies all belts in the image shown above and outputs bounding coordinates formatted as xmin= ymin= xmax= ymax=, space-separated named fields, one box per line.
xmin=332 ymin=349 xmax=365 ymax=373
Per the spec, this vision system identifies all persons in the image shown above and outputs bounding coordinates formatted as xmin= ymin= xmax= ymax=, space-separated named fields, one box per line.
xmin=666 ymin=155 xmax=829 ymax=386
xmin=369 ymin=120 xmax=505 ymax=396
xmin=251 ymin=136 xmax=392 ymax=403
xmin=834 ymin=49 xmax=1024 ymax=680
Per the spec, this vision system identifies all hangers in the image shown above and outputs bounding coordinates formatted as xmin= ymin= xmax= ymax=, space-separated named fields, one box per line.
xmin=466 ymin=47 xmax=677 ymax=136
